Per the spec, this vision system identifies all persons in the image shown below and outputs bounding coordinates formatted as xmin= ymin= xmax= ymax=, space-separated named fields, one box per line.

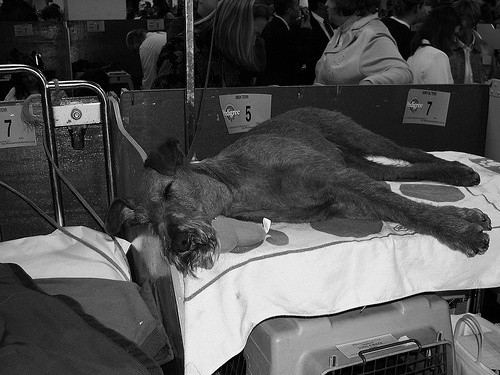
xmin=0 ymin=0 xmax=500 ymax=86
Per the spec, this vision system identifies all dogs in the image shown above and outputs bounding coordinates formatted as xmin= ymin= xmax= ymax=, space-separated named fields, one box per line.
xmin=102 ymin=107 xmax=492 ymax=279
